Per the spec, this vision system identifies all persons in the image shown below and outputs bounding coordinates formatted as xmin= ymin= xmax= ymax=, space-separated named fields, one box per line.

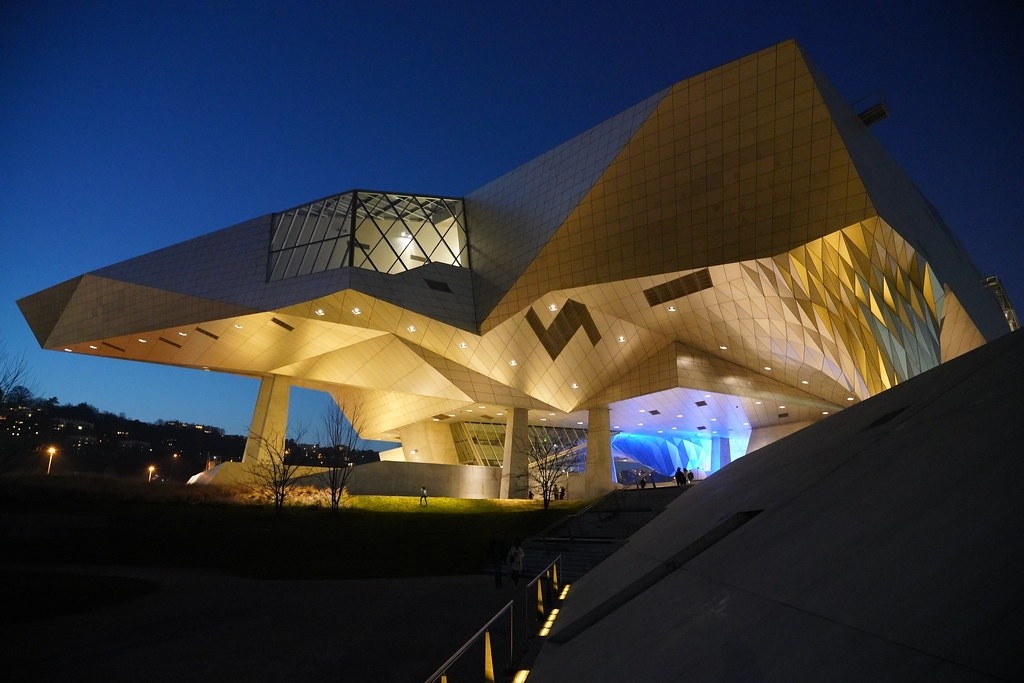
xmin=486 ymin=531 xmax=508 ymax=589
xmin=675 ymin=467 xmax=694 ymax=486
xmin=506 ymin=536 xmax=526 ymax=587
xmin=639 ymin=478 xmax=645 ymax=489
xmin=553 ymin=483 xmax=565 ymax=500
xmin=419 ymin=487 xmax=428 ymax=507
xmin=529 ymin=491 xmax=534 ymax=499
